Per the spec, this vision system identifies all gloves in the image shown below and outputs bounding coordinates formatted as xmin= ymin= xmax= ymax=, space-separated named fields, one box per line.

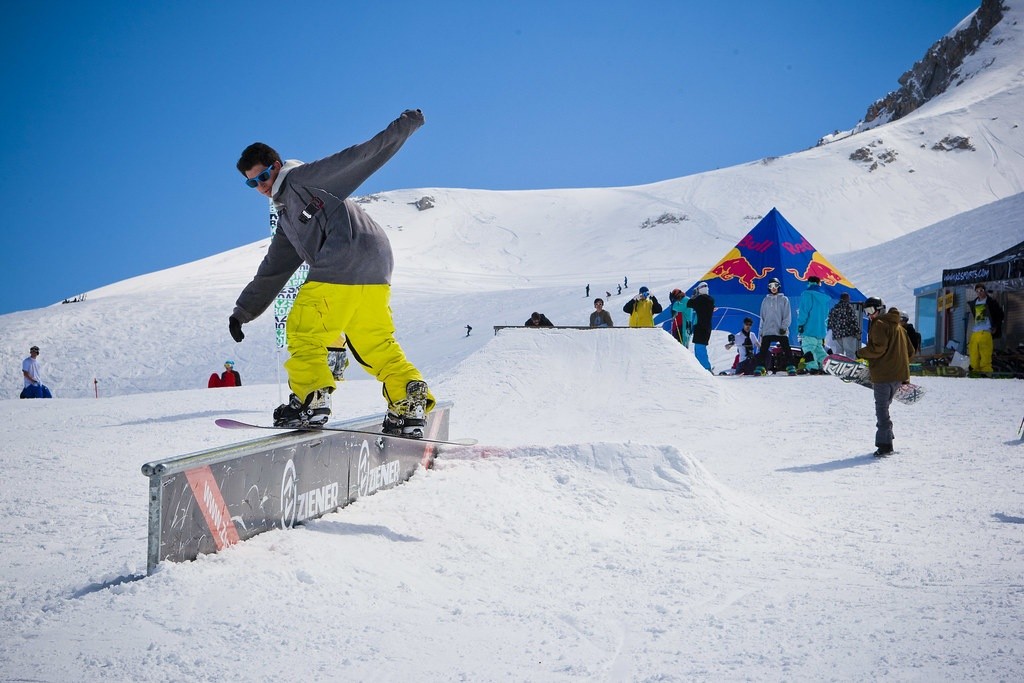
xmin=798 ymin=326 xmax=804 ymax=333
xmin=229 ymin=317 xmax=244 ymax=342
xmin=780 ymin=329 xmax=786 ymax=334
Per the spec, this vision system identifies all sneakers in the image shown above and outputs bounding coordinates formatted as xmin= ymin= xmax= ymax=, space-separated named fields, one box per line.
xmin=383 ymin=380 xmax=428 ymax=433
xmin=873 ymin=443 xmax=893 ymax=456
xmin=754 ymin=366 xmax=766 ymax=376
xmin=786 ymin=365 xmax=798 ymax=376
xmin=273 ymin=388 xmax=332 ymax=425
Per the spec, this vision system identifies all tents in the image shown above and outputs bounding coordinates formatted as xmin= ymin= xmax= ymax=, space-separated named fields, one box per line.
xmin=654 ymin=206 xmax=869 ymax=346
xmin=941 ymin=241 xmax=1024 ymax=353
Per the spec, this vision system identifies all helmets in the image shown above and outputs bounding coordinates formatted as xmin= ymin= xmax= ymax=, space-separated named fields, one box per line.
xmin=865 ymin=297 xmax=886 ymax=313
xmin=769 ymin=278 xmax=781 ymax=290
xmin=900 ymin=311 xmax=909 ymax=319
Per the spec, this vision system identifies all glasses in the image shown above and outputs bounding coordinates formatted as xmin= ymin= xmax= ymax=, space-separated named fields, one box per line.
xmin=769 ymin=283 xmax=779 ymax=289
xmin=642 ymin=292 xmax=650 ymax=296
xmin=976 ymin=289 xmax=984 ymax=293
xmin=224 ymin=364 xmax=231 ymax=367
xmin=245 ymin=163 xmax=274 ymax=188
xmin=864 ymin=306 xmax=876 ymax=316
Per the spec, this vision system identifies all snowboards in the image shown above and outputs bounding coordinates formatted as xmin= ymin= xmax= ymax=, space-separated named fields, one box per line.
xmin=215 ymin=418 xmax=479 ymax=446
xmin=822 ymin=353 xmax=927 ymax=406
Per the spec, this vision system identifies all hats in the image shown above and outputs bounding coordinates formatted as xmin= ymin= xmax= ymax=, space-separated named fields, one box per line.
xmin=640 ymin=287 xmax=648 ymax=294
xmin=672 ymin=288 xmax=685 ymax=299
xmin=226 ymin=360 xmax=234 ymax=367
xmin=698 ymin=282 xmax=708 ymax=294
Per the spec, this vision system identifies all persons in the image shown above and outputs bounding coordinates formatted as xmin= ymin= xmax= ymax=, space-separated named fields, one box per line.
xmin=466 ymin=325 xmax=472 ymax=335
xmin=798 ymin=276 xmax=829 ymax=375
xmin=671 ymin=289 xmax=693 ymax=348
xmin=228 ymin=108 xmax=435 ymax=439
xmin=624 ymin=276 xmax=628 ymax=287
xmin=899 ymin=311 xmax=915 ymax=337
xmin=586 ymin=284 xmax=589 ymax=297
xmin=855 ymin=297 xmax=910 ymax=456
xmin=589 ymin=298 xmax=614 ymax=327
xmin=623 ymin=286 xmax=663 ymax=327
xmin=735 ymin=317 xmax=761 ymax=363
xmin=827 ymin=292 xmax=862 ymax=360
xmin=618 ymin=284 xmax=622 ymax=294
xmin=525 ymin=311 xmax=554 ymax=326
xmin=754 ymin=278 xmax=797 ymax=376
xmin=687 ymin=282 xmax=715 ymax=370
xmin=22 ymin=346 xmax=41 ymax=399
xmin=222 ymin=361 xmax=241 ymax=386
xmin=968 ymin=284 xmax=1006 ymax=373
